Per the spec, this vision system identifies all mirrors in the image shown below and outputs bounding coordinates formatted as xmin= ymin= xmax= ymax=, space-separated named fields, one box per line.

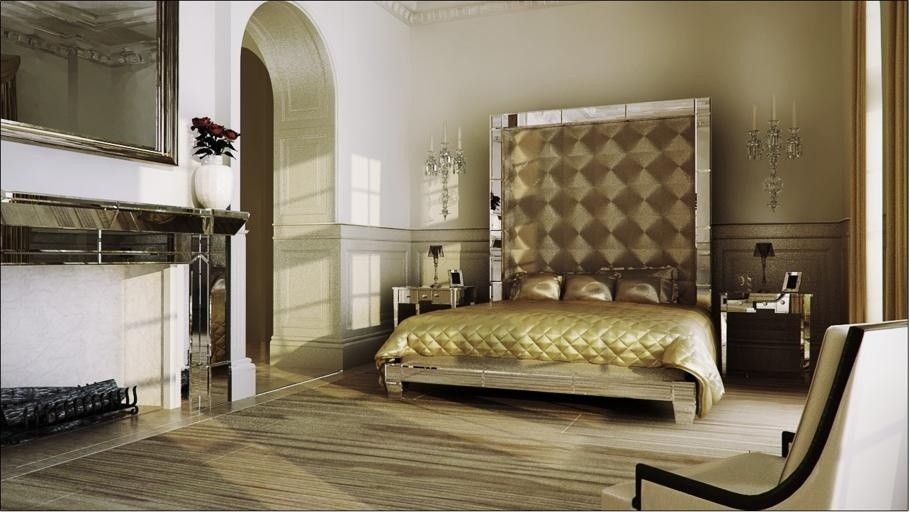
xmin=1 ymin=1 xmax=180 ymax=165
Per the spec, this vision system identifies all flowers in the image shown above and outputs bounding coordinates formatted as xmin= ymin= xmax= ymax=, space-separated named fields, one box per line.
xmin=189 ymin=117 xmax=241 ymax=160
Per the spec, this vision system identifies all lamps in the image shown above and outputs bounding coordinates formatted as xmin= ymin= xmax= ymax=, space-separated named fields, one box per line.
xmin=427 ymin=245 xmax=444 ymax=287
xmin=754 ymin=243 xmax=775 ymax=293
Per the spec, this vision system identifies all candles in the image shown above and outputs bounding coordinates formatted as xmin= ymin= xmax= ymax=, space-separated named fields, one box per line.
xmin=457 ymin=129 xmax=462 ymax=149
xmin=771 ymin=94 xmax=777 ymax=119
xmin=752 ymin=104 xmax=757 ymax=131
xmin=428 ymin=133 xmax=433 ymax=151
xmin=791 ymin=102 xmax=797 ymax=128
xmin=443 ymin=120 xmax=446 ymax=142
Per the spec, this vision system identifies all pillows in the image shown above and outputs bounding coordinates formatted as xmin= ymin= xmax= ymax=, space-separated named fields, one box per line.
xmin=563 ymin=274 xmax=617 ymax=302
xmin=596 ymin=266 xmax=678 ymax=305
xmin=509 ymin=270 xmax=562 ymax=302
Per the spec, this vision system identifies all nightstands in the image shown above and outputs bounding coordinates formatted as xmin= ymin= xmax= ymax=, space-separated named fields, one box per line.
xmin=719 ymin=291 xmax=813 ymax=387
xmin=391 ymin=287 xmax=477 ymax=332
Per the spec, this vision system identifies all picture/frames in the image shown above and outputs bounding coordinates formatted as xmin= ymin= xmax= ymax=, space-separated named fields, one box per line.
xmin=448 ymin=269 xmax=464 ymax=287
xmin=781 ymin=271 xmax=803 ymax=292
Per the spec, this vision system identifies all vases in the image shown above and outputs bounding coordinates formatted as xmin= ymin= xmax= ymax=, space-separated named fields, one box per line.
xmin=195 ymin=155 xmax=233 ymax=211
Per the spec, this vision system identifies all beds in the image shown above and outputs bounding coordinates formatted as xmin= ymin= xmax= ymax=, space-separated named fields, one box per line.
xmin=374 ymin=98 xmax=725 ymax=424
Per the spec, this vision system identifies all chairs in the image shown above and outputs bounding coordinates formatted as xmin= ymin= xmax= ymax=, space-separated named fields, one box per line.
xmin=600 ymin=318 xmax=908 ymax=512
